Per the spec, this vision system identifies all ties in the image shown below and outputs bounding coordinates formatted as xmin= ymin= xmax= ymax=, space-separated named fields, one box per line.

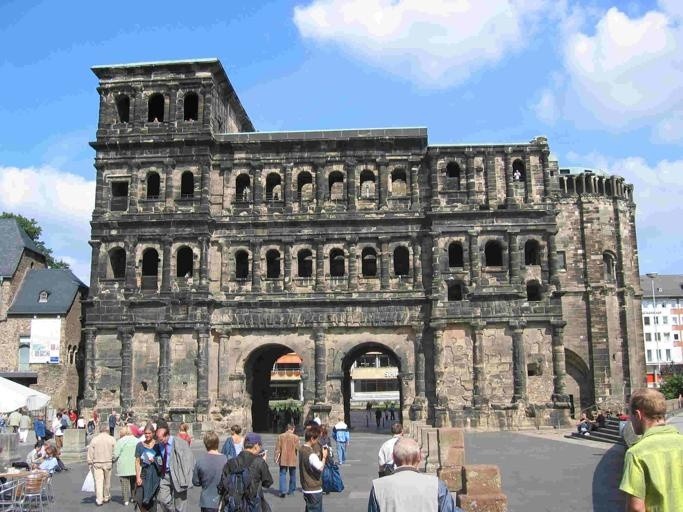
xmin=161 ymin=443 xmax=168 ymax=479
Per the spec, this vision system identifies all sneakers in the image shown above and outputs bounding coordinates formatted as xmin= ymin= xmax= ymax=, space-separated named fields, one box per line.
xmin=95 ymin=499 xmax=110 ymax=507
xmin=122 ymin=498 xmax=134 ymax=507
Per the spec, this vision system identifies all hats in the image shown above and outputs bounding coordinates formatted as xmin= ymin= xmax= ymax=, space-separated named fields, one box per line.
xmin=243 ymin=433 xmax=262 ymax=447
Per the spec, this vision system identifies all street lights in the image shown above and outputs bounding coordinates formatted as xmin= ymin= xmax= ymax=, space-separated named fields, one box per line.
xmin=645 ymin=270 xmax=663 ymax=375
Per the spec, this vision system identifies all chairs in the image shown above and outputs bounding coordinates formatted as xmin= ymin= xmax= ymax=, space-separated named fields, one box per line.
xmin=0 ymin=462 xmax=56 ymax=511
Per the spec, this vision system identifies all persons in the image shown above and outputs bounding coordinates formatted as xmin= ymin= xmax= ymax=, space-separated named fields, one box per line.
xmin=618 ymin=388 xmax=683 ymax=512
xmin=576 ymin=402 xmax=624 ymax=435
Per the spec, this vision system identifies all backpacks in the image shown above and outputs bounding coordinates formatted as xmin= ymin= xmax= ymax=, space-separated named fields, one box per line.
xmin=216 ymin=458 xmax=262 ymax=512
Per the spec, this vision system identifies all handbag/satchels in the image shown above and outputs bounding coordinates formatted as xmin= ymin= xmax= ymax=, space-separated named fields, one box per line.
xmin=319 ymin=452 xmax=344 ymax=494
